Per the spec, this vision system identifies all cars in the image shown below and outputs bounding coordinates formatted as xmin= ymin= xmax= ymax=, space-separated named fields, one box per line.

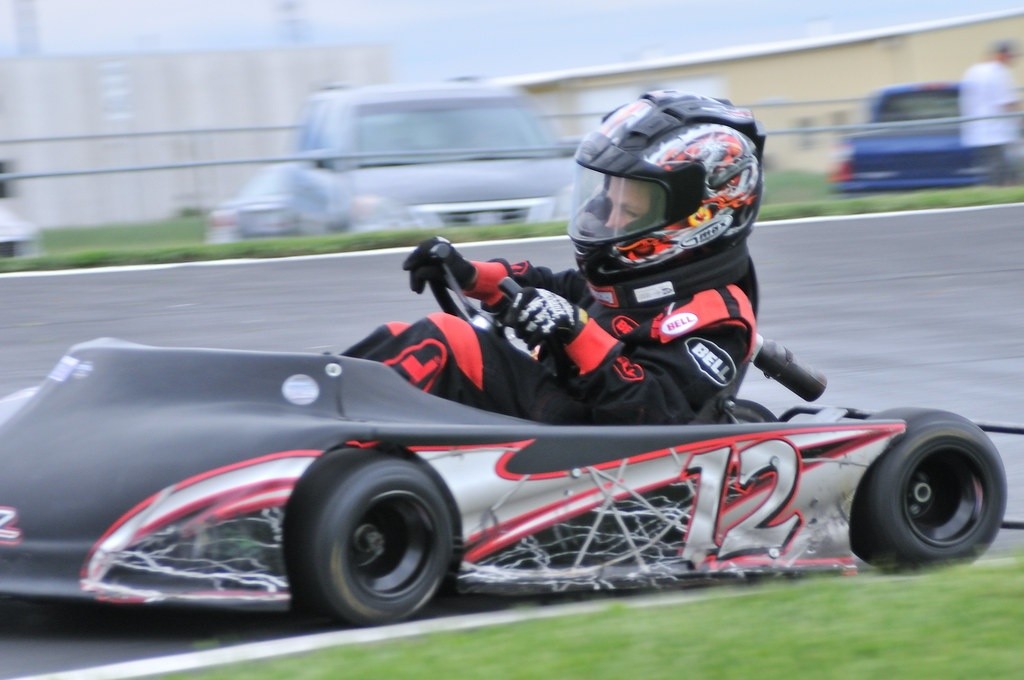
xmin=827 ymin=78 xmax=1010 ymax=197
xmin=279 ymin=79 xmax=585 ymax=242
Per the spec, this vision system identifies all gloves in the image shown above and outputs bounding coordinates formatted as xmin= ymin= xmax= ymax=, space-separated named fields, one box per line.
xmin=506 ymin=287 xmax=588 ymax=351
xmin=404 ymin=236 xmax=475 ymax=294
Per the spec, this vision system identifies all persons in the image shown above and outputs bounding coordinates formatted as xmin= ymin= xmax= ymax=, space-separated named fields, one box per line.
xmin=339 ymin=89 xmax=766 ymax=425
xmin=959 ymin=38 xmax=1023 ymax=187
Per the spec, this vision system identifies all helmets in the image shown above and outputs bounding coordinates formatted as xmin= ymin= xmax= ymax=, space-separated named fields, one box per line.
xmin=565 ymin=89 xmax=766 ymax=306
